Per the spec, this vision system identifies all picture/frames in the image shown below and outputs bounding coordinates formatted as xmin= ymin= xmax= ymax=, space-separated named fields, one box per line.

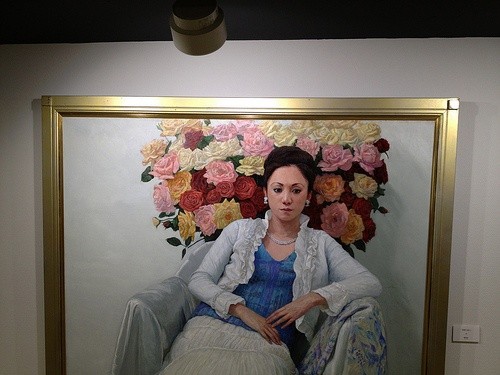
xmin=40 ymin=96 xmax=460 ymax=375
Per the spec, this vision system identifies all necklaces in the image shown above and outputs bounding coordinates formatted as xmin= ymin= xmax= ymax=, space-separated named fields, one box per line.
xmin=266 ymin=230 xmax=297 ymax=246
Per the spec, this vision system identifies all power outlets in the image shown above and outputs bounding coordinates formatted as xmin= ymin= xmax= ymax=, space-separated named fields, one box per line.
xmin=452 ymin=324 xmax=480 ymax=343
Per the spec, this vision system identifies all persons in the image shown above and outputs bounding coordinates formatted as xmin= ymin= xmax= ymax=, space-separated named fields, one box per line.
xmin=155 ymin=145 xmax=382 ymax=375
